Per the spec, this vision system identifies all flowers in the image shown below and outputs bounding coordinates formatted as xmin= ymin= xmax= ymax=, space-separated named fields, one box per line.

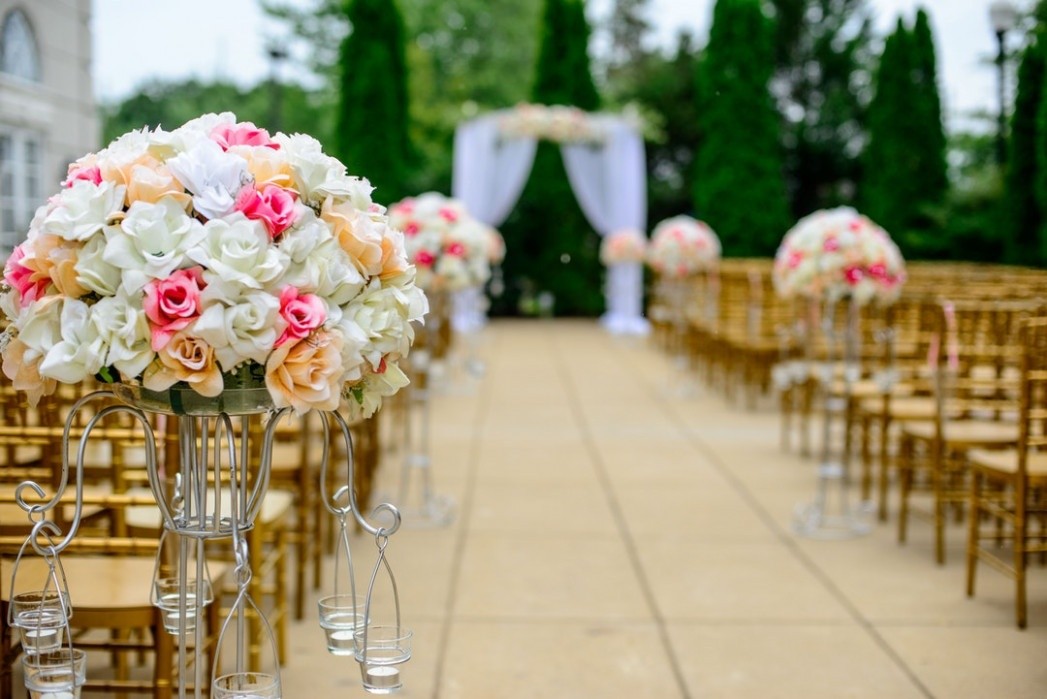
xmin=599 ymin=216 xmax=721 ymax=275
xmin=772 ymin=203 xmax=906 ymax=311
xmin=0 ymin=111 xmax=506 ymax=427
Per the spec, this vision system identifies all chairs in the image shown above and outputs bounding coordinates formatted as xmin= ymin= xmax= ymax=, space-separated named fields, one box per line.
xmin=0 ymin=298 xmax=450 ymax=699
xmin=650 ymin=260 xmax=1047 ymax=630
